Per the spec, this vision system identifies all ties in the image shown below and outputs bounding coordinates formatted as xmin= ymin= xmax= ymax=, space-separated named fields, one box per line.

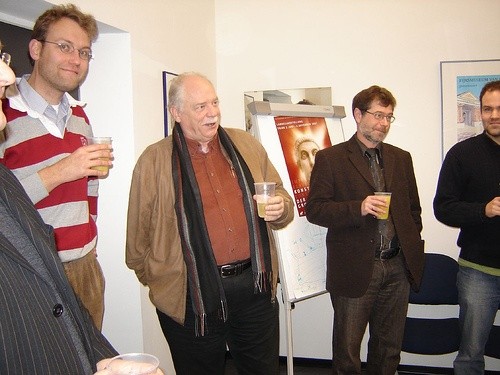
xmin=366 ymin=148 xmax=395 ymax=241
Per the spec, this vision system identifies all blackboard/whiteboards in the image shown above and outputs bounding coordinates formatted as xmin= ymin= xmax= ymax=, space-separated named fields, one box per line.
xmin=249 ymin=101 xmax=347 ymax=303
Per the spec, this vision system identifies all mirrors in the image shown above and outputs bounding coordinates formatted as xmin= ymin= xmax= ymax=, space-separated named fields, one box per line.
xmin=244 ymin=87 xmax=331 ymax=135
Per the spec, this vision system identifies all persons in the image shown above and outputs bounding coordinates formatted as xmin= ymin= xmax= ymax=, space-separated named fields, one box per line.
xmin=125 ymin=73 xmax=294 ymax=375
xmin=307 ymin=85 xmax=425 ymax=375
xmin=0 ymin=3 xmax=113 ymax=333
xmin=0 ymin=48 xmax=164 ymax=375
xmin=433 ymin=80 xmax=500 ymax=375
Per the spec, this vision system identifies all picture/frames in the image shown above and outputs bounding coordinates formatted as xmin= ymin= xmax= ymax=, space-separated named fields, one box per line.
xmin=162 ymin=71 xmax=178 ymax=137
xmin=440 ymin=59 xmax=500 ymax=162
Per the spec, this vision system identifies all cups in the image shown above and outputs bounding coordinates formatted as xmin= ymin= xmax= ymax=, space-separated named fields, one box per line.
xmin=373 ymin=192 xmax=392 ymax=219
xmin=85 ymin=136 xmax=113 ymax=174
xmin=106 ymin=353 xmax=159 ymax=375
xmin=254 ymin=182 xmax=277 ymax=218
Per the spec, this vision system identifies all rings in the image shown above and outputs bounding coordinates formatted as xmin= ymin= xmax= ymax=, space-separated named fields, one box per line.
xmin=371 ymin=206 xmax=375 ymax=211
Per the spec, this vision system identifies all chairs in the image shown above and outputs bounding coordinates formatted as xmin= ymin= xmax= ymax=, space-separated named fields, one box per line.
xmin=401 ymin=252 xmax=500 ymax=359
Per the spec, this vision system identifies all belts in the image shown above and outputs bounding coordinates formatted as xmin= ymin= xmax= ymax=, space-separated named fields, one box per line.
xmin=217 ymin=261 xmax=251 ymax=279
xmin=375 ymin=246 xmax=400 ymax=261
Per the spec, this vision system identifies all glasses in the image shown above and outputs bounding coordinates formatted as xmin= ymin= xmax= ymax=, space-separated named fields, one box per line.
xmin=0 ymin=49 xmax=11 ymax=66
xmin=360 ymin=111 xmax=395 ymax=122
xmin=42 ymin=40 xmax=92 ymax=60
